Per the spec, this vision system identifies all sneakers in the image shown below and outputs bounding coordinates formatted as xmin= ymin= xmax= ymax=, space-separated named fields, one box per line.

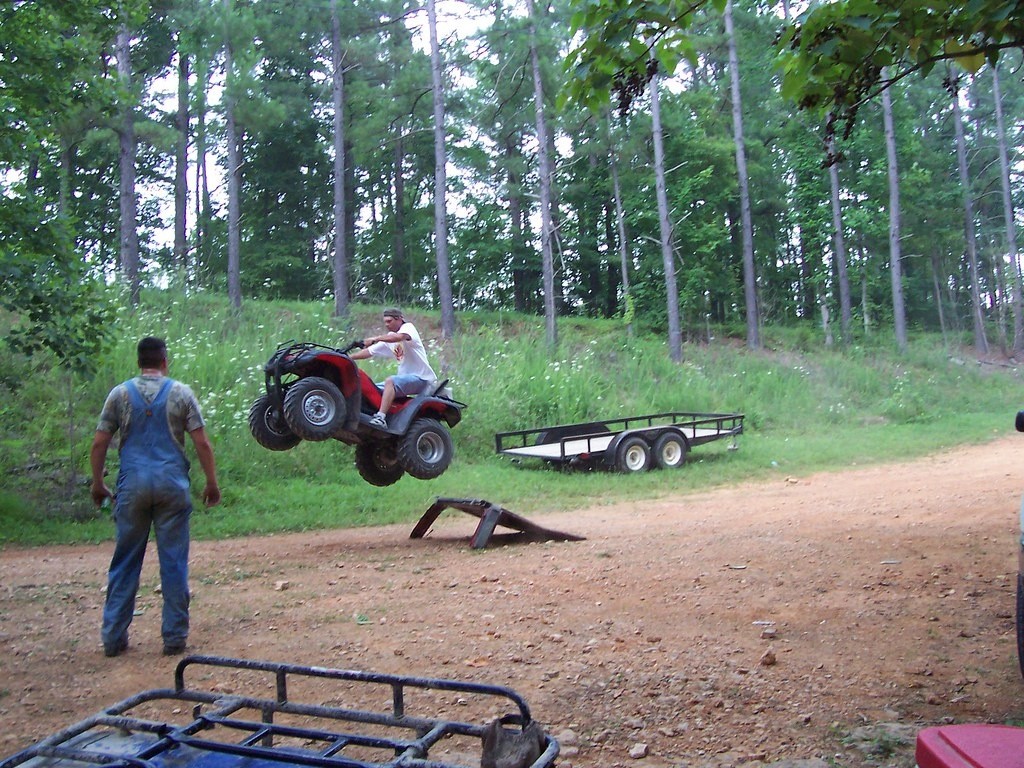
xmin=369 ymin=417 xmax=389 ymax=429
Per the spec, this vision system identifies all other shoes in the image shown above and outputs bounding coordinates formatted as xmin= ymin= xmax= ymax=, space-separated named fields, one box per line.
xmin=105 ymin=641 xmax=128 ymax=655
xmin=163 ymin=642 xmax=186 ymax=654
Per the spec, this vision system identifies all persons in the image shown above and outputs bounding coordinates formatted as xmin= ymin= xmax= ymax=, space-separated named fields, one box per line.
xmin=348 ymin=309 xmax=436 ymax=430
xmin=92 ymin=338 xmax=221 ymax=656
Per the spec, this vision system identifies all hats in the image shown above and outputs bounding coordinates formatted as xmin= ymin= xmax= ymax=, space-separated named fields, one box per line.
xmin=384 ymin=308 xmax=406 ymax=323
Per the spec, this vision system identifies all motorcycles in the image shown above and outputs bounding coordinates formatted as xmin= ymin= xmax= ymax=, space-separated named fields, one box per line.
xmin=249 ymin=337 xmax=468 ymax=488
